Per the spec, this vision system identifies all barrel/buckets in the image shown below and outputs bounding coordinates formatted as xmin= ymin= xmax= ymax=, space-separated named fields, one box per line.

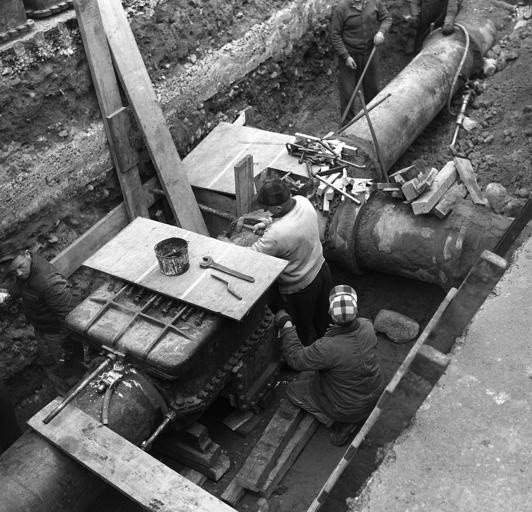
xmin=154 ymin=236 xmax=189 ymax=275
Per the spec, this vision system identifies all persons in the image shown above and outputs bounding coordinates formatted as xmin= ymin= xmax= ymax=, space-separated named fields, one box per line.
xmin=329 ymin=0 xmax=394 ymax=129
xmin=215 ymin=179 xmax=326 ymax=345
xmin=272 ymin=283 xmax=381 ymax=446
xmin=409 ymin=0 xmax=460 ymax=55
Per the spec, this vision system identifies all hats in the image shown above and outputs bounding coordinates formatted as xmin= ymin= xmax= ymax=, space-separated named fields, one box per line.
xmin=257 ymin=179 xmax=290 ymax=206
xmin=0 ymin=237 xmax=33 ymax=263
xmin=328 ymin=284 xmax=358 ymax=324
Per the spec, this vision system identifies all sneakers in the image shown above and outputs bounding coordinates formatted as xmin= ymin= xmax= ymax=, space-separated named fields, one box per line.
xmin=330 ymin=421 xmax=363 ymax=447
